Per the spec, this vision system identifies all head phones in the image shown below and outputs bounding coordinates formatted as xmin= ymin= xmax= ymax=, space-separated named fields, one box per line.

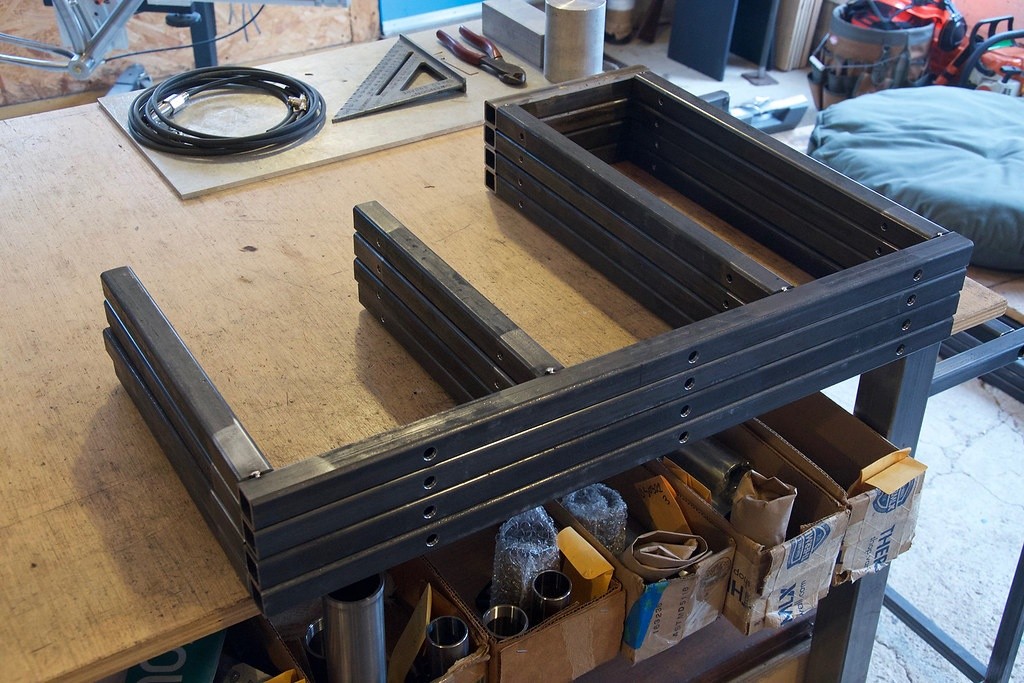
xmin=911 ymin=0 xmax=968 ymax=52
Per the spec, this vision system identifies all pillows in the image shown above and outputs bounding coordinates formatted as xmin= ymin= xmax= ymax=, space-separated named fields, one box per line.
xmin=809 ymin=84 xmax=1024 ymax=269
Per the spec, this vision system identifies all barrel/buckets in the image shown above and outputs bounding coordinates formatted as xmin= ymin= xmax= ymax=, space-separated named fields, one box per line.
xmin=810 ymin=3 xmax=935 ymax=112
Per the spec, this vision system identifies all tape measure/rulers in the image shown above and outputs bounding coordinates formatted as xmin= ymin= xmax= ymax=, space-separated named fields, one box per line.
xmin=329 ymin=33 xmax=466 ymax=124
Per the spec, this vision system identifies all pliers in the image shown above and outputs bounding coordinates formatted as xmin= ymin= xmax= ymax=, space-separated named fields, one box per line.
xmin=436 ymin=25 xmax=527 ymax=86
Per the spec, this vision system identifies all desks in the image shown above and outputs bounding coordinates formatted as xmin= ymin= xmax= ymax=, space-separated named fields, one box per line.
xmin=0 ymin=20 xmax=1007 ymax=683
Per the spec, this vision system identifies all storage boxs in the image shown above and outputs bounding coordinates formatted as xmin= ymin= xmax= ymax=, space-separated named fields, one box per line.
xmin=647 ymin=424 xmax=851 ymax=636
xmin=426 ymin=505 xmax=626 ymax=683
xmin=744 ymin=390 xmax=927 ymax=583
xmin=544 ymin=460 xmax=736 ymax=667
xmin=253 ymin=556 xmax=490 ymax=683
